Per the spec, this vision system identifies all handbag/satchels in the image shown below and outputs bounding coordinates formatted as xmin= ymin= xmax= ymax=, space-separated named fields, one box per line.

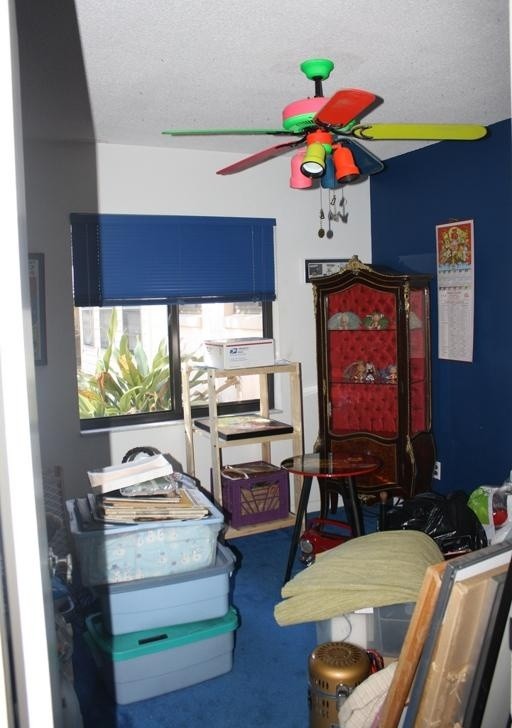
xmin=119 ymin=447 xmax=178 ymax=497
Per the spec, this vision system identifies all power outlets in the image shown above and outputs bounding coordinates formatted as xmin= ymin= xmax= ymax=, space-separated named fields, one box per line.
xmin=432 ymin=461 xmax=441 ymax=480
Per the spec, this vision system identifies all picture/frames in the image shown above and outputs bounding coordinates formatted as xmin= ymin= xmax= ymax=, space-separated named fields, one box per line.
xmin=28 ymin=253 xmax=49 ymax=366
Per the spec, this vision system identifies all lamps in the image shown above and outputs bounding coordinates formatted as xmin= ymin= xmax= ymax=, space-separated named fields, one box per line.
xmin=289 ymin=141 xmax=360 ymax=189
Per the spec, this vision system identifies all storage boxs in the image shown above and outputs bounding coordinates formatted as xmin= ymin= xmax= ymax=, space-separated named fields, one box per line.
xmin=201 ymin=336 xmax=276 ymax=371
xmin=209 ymin=461 xmax=290 ymax=531
xmin=64 ymin=487 xmax=241 ymax=707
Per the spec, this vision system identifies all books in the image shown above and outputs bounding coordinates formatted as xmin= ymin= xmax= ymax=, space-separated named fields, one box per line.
xmin=73 ymin=452 xmax=211 ymax=531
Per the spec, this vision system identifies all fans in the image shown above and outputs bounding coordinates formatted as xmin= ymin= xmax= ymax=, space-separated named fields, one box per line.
xmin=162 ymin=60 xmax=487 ymax=239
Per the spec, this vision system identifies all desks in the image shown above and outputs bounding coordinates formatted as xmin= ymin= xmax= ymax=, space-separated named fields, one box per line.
xmin=280 ymin=451 xmax=384 ymax=586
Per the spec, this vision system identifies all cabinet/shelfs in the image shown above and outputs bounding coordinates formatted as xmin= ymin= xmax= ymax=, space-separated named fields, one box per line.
xmin=180 ymin=358 xmax=306 ymax=545
xmin=310 ymin=255 xmax=435 ymax=529
